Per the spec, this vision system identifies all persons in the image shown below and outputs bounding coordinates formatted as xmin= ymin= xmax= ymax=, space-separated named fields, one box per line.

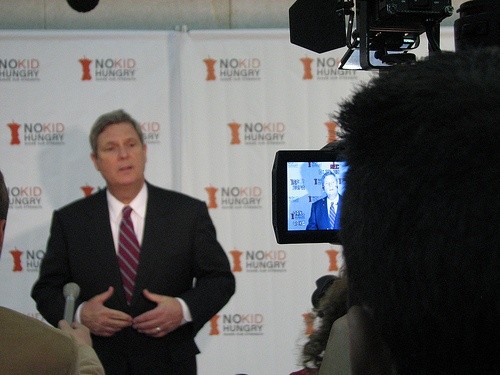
xmin=306 ymin=171 xmax=343 ymax=230
xmin=31 ymin=109 xmax=235 ymax=375
xmin=0 ymin=170 xmax=106 ymax=375
xmin=331 ymin=45 xmax=500 ymax=375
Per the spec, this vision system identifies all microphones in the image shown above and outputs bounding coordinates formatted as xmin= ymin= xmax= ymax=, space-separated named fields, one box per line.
xmin=62 ymin=283 xmax=81 ymax=327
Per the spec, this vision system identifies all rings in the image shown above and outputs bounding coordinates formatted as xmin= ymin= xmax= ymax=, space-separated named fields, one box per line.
xmin=157 ymin=326 xmax=161 ymax=331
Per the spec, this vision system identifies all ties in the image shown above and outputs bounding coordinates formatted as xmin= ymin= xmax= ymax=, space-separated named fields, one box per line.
xmin=329 ymin=202 xmax=336 ymax=229
xmin=117 ymin=206 xmax=142 ymax=305
